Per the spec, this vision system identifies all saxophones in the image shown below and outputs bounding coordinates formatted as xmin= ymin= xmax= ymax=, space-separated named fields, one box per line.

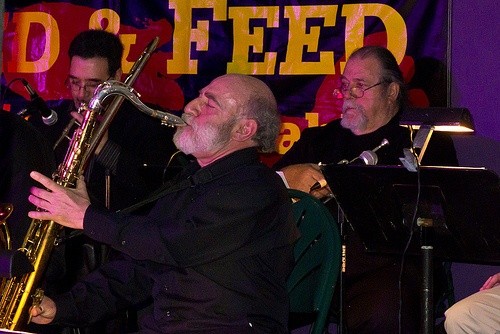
xmin=0 ymin=35 xmax=188 ymax=334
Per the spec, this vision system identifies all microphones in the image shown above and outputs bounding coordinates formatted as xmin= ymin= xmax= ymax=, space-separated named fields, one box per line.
xmin=310 ymin=150 xmax=378 ymax=191
xmin=22 ymin=78 xmax=58 ymax=126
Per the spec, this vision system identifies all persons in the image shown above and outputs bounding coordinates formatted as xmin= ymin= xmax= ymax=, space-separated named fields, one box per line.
xmin=267 ymin=46 xmax=463 ymax=334
xmin=26 ymin=72 xmax=296 ymax=334
xmin=1 ymin=30 xmax=176 ymax=334
xmin=442 ymin=271 xmax=500 ymax=334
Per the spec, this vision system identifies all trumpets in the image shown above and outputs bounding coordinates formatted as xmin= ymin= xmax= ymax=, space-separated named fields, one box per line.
xmin=292 ymin=138 xmax=390 ymax=203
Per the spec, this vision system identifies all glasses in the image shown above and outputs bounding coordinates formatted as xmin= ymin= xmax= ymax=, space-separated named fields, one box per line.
xmin=64 ymin=70 xmax=116 ymax=93
xmin=331 ymin=80 xmax=392 ymax=101
